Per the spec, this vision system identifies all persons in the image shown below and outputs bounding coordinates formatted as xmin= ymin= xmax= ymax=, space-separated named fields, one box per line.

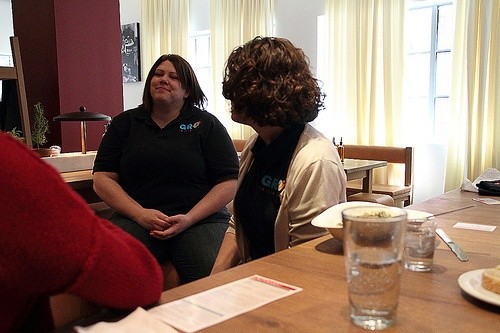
xmin=209 ymin=37 xmax=347 ymax=276
xmin=91 ymin=54 xmax=240 ymax=282
xmin=0 ymin=132 xmax=163 ymax=333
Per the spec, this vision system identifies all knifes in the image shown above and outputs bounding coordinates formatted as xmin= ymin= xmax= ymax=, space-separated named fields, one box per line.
xmin=436 ymin=228 xmax=469 ymax=262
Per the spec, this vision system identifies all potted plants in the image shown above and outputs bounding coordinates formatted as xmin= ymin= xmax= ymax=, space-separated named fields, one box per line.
xmin=28 ymin=101 xmax=52 ymax=157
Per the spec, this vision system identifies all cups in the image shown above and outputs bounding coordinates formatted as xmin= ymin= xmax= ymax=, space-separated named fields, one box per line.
xmin=405 ymin=218 xmax=438 ymax=272
xmin=49 ymin=146 xmax=60 ymax=157
xmin=341 ymin=205 xmax=408 ymax=331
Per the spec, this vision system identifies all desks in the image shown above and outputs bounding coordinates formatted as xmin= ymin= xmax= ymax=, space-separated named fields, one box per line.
xmin=339 ymin=158 xmax=388 ymax=198
xmin=55 ymin=181 xmax=500 ymax=333
xmin=56 ymin=170 xmax=121 ymax=220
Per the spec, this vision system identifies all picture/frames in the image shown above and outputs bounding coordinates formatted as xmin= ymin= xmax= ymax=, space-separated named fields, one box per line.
xmin=120 ymin=21 xmax=141 ymax=84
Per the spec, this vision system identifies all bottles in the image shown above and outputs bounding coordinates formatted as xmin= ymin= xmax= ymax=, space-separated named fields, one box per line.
xmin=337 ymin=136 xmax=344 ymax=164
xmin=332 ymin=136 xmax=335 ymax=145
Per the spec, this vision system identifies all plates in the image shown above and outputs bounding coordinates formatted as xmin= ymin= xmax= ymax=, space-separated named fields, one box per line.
xmin=403 ymin=209 xmax=437 ymax=223
xmin=457 ymin=264 xmax=500 ymax=306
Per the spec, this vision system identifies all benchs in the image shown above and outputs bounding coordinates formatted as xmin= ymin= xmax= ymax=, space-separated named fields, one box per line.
xmin=233 ymin=138 xmax=414 ymax=208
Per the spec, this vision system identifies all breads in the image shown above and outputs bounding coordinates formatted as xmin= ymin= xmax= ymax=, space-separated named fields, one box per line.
xmin=482 ymin=265 xmax=500 ymax=294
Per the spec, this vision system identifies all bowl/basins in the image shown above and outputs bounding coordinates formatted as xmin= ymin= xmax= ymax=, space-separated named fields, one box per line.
xmin=311 ymin=201 xmax=389 ymax=241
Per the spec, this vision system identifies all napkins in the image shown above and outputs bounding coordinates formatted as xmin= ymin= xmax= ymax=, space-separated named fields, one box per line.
xmin=459 ymin=167 xmax=500 ymax=192
xmin=73 ymin=303 xmax=176 ymax=333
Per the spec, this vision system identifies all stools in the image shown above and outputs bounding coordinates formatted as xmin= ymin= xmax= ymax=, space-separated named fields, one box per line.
xmin=348 ymin=192 xmax=395 ymax=207
